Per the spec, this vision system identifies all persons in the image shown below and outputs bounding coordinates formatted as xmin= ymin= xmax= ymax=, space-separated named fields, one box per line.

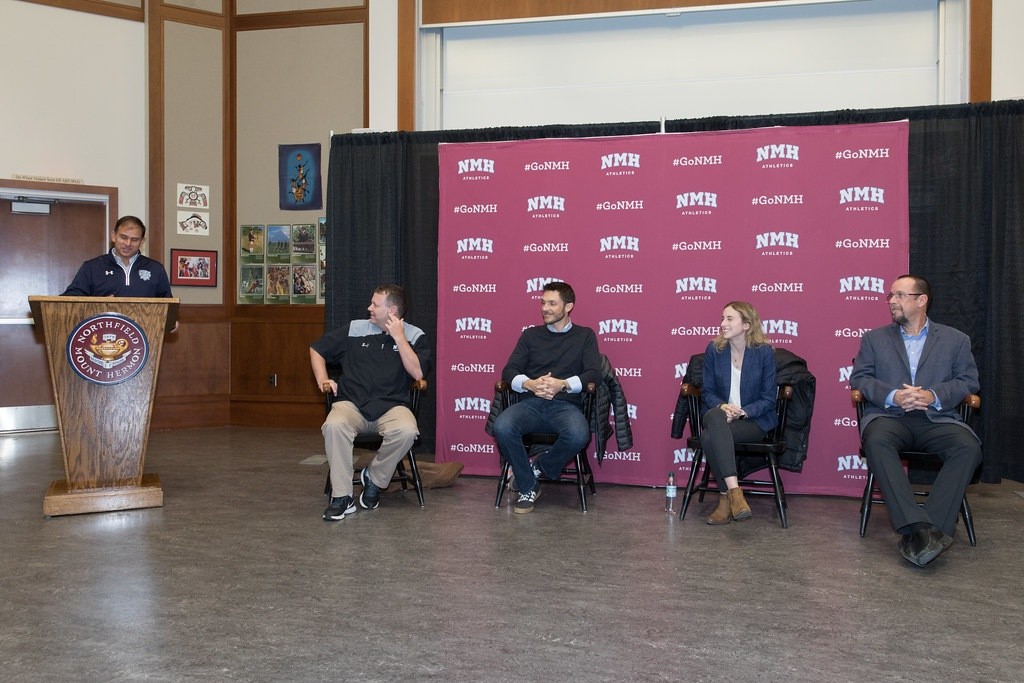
xmin=493 ymin=281 xmax=603 ymax=515
xmin=310 ymin=283 xmax=432 ymax=521
xmin=294 ymin=267 xmax=316 ymax=293
xmin=848 ymin=276 xmax=983 ymax=567
xmin=251 ymin=277 xmax=258 ymax=289
xmin=248 ymin=229 xmax=256 ymax=253
xmin=278 ymin=239 xmax=287 ymax=248
xmin=299 ymin=228 xmax=308 ymax=251
xmin=179 ymin=258 xmax=208 ymax=278
xmin=701 ymin=302 xmax=778 ymax=524
xmin=267 ymin=266 xmax=289 ymax=294
xmin=58 ymin=216 xmax=180 ymax=333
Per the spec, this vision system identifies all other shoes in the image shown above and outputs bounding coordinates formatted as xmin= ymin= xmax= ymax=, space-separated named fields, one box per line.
xmin=726 ymin=487 xmax=752 ymax=520
xmin=913 ymin=526 xmax=953 ymax=565
xmin=706 ymin=493 xmax=733 ymax=525
xmin=898 ymin=532 xmax=925 ymax=568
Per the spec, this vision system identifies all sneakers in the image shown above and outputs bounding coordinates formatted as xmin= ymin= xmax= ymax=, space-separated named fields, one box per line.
xmin=514 ymin=481 xmax=542 ymax=514
xmin=509 ymin=464 xmax=542 ymax=491
xmin=359 ymin=466 xmax=380 ymax=509
xmin=322 ymin=495 xmax=357 ymax=520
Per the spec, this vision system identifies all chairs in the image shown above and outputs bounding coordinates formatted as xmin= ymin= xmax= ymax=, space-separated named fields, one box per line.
xmin=670 ymin=347 xmax=817 ymax=528
xmin=322 ymin=361 xmax=425 ymax=507
xmin=850 ymin=358 xmax=981 ymax=548
xmin=484 ymin=353 xmax=633 ymax=513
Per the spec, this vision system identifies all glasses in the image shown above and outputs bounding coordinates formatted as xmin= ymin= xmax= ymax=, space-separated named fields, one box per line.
xmin=886 ymin=292 xmax=922 ymax=300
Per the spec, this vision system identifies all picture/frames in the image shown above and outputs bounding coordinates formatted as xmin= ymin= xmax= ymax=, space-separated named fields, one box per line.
xmin=169 ymin=247 xmax=218 ymax=287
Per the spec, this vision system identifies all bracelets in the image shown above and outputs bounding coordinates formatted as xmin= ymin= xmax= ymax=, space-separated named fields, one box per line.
xmin=719 ymin=402 xmax=727 ymax=408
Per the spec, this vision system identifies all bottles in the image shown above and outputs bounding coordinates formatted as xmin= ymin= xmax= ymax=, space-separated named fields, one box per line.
xmin=666 ymin=472 xmax=677 ymax=512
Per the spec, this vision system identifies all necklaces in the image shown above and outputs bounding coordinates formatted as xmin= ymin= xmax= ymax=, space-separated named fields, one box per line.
xmin=734 ymin=358 xmax=738 ymax=362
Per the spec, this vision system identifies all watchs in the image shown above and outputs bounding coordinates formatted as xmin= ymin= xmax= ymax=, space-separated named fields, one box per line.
xmin=561 ymin=380 xmax=566 ymax=392
xmin=738 ymin=414 xmax=745 ymax=420
